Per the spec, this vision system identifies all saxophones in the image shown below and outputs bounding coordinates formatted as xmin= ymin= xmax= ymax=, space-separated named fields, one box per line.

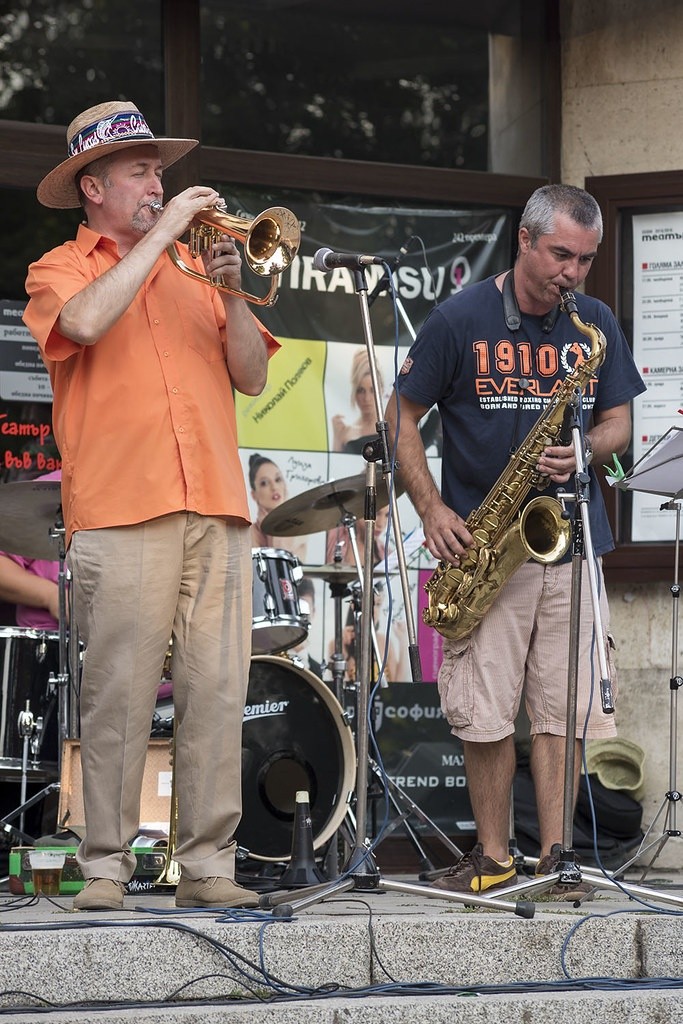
xmin=422 ymin=286 xmax=607 ymax=642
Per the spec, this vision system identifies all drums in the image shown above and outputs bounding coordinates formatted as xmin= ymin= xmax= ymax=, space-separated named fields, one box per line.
xmin=236 ymin=656 xmax=357 ymax=861
xmin=0 ymin=625 xmax=86 ymax=784
xmin=252 ymin=547 xmax=309 ymax=655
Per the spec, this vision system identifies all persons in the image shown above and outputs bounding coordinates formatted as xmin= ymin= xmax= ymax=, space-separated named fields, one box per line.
xmin=332 ymin=349 xmax=385 ymax=451
xmin=23 ymin=103 xmax=283 ymax=907
xmin=283 ymin=578 xmax=323 ymax=679
xmin=328 ymin=581 xmax=410 ymax=683
xmin=0 ymin=469 xmax=176 ymax=700
xmin=383 ymin=185 xmax=648 ymax=900
xmin=246 ymin=453 xmax=308 ymax=564
xmin=327 ymin=468 xmax=395 ymax=567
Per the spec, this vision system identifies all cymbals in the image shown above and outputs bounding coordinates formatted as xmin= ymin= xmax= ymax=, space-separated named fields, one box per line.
xmin=0 ymin=481 xmax=67 ymax=561
xmin=301 ymin=565 xmax=399 ymax=582
xmin=261 ymin=469 xmax=406 ymax=538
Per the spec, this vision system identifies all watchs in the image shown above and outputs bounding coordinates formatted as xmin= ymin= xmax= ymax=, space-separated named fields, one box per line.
xmin=582 ymin=435 xmax=593 ymax=467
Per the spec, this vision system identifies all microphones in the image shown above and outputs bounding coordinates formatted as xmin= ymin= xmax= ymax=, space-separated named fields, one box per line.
xmin=313 ymin=247 xmax=384 ymax=272
xmin=557 ymin=388 xmax=580 ymax=447
xmin=368 ymin=236 xmax=415 ymax=306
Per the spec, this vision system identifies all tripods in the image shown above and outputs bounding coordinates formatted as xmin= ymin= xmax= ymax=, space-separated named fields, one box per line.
xmin=259 ymin=267 xmax=683 ymax=924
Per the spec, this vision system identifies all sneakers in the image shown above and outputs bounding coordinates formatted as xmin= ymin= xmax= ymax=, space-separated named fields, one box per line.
xmin=534 ymin=844 xmax=594 ymax=900
xmin=74 ymin=878 xmax=128 ymax=909
xmin=176 ymin=876 xmax=259 ymax=908
xmin=429 ymin=842 xmax=518 ymax=894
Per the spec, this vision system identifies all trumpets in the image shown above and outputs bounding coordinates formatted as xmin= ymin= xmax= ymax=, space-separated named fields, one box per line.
xmin=148 ymin=199 xmax=301 ymax=308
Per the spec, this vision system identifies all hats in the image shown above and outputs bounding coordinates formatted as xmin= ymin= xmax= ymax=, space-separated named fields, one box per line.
xmin=37 ymin=101 xmax=199 ymax=210
xmin=581 ymin=736 xmax=646 ymax=800
